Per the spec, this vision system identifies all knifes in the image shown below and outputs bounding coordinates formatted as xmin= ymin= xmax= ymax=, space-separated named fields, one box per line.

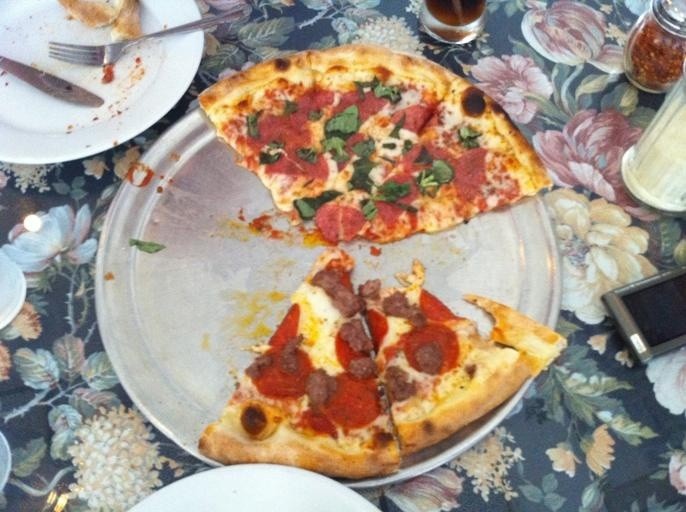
xmin=1 ymin=56 xmax=103 ymax=110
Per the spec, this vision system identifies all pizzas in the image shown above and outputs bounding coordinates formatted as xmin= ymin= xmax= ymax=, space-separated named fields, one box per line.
xmin=198 ymin=44 xmax=568 ymax=474
xmin=56 ymin=0 xmax=140 ymax=40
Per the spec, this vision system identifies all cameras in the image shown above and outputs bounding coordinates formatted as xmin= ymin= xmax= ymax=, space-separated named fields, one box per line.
xmin=599 ymin=265 xmax=686 ymax=367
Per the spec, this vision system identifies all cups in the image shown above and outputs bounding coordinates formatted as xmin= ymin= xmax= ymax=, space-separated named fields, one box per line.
xmin=620 ymin=64 xmax=685 ymax=211
xmin=414 ymin=0 xmax=488 ymax=45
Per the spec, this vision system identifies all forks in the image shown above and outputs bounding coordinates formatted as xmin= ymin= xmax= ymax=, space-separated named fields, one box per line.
xmin=49 ymin=3 xmax=255 ymax=68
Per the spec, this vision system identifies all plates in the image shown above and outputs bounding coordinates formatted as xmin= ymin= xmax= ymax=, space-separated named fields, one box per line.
xmin=0 ymin=257 xmax=26 ymax=330
xmin=92 ymin=48 xmax=559 ymax=494
xmin=0 ymin=1 xmax=205 ymax=166
xmin=126 ymin=464 xmax=385 ymax=512
xmin=0 ymin=434 xmax=12 ymax=493
